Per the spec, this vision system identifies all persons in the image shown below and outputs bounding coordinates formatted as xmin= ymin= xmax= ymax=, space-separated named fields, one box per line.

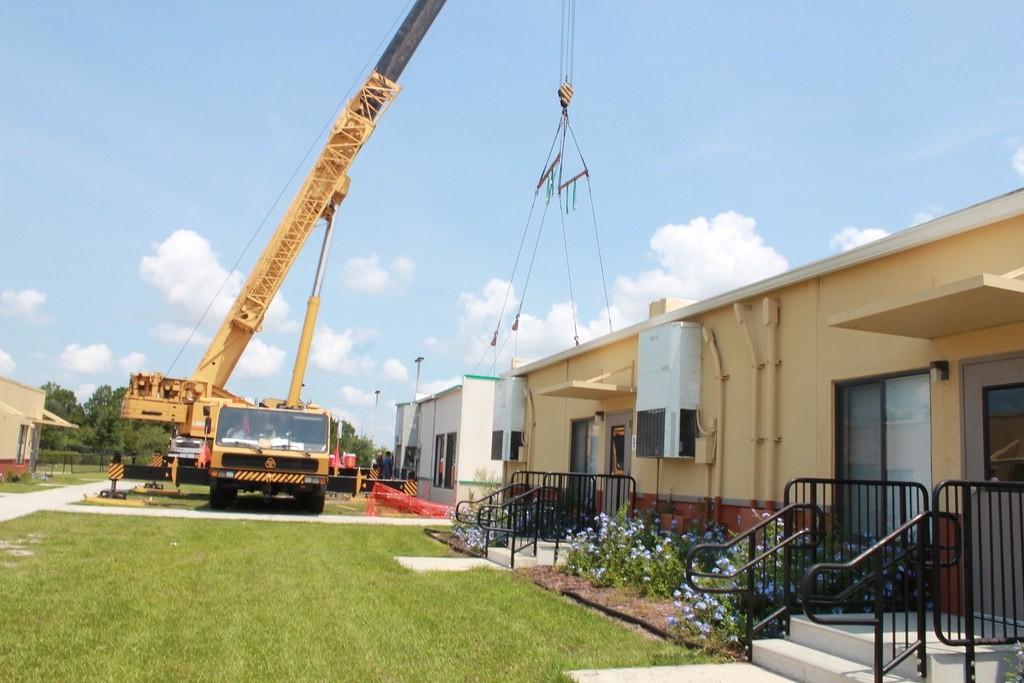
xmin=382 ymin=451 xmax=392 ymax=479
xmin=226 ymin=421 xmax=247 ymax=440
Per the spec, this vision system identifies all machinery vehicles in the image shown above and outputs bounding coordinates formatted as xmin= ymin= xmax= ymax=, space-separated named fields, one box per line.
xmin=97 ymin=0 xmax=615 ymax=515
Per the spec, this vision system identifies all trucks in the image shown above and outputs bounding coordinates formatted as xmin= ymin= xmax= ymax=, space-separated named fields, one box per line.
xmin=162 ymin=430 xmax=203 ymax=467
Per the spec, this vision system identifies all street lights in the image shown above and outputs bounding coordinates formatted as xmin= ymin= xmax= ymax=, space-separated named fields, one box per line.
xmin=369 ymin=389 xmax=382 ymax=469
xmin=415 ymin=358 xmax=425 ymax=400
